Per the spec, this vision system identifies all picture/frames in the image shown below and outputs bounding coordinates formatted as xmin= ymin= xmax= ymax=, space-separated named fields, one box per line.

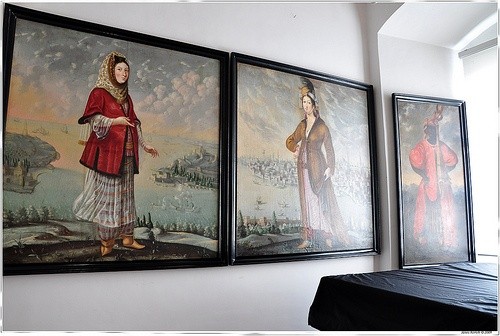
xmin=229 ymin=50 xmax=384 ymax=266
xmin=0 ymin=2 xmax=230 ymax=277
xmin=392 ymin=91 xmax=478 ymax=269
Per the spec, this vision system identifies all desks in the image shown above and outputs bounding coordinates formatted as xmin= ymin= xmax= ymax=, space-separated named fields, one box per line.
xmin=305 ymin=261 xmax=498 ymax=333
